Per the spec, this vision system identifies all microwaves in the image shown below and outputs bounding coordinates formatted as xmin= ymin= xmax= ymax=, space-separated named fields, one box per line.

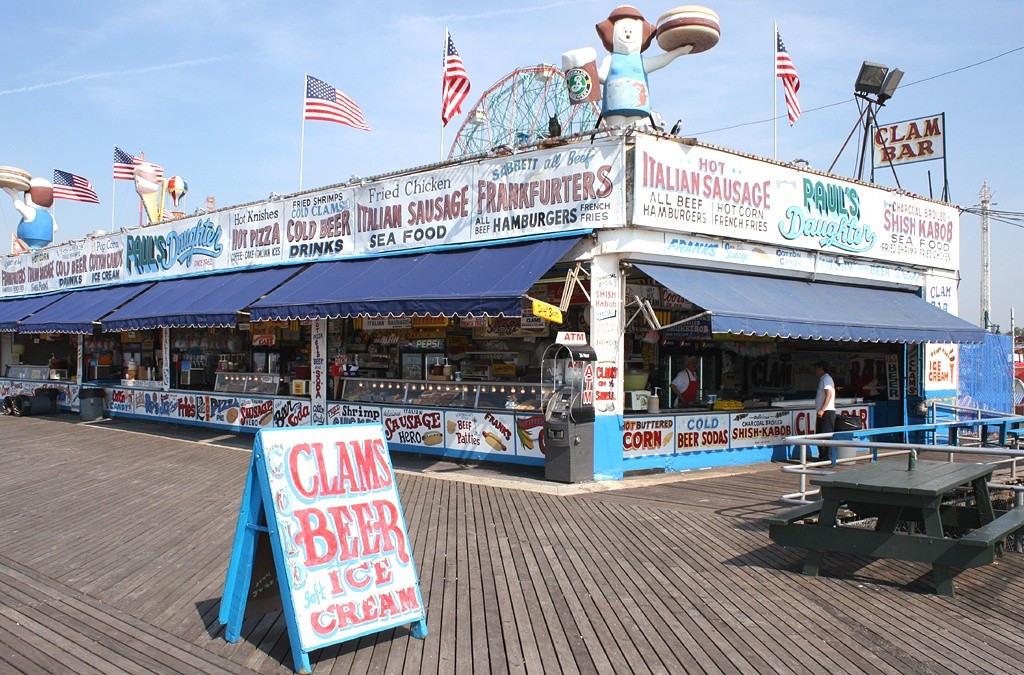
xmin=292 ymin=379 xmax=310 ymax=395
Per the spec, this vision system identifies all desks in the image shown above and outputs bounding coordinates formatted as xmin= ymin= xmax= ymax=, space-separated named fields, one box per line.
xmin=802 ymin=456 xmax=1002 ymax=597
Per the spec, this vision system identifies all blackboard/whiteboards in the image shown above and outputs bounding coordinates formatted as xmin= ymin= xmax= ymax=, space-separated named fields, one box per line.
xmin=217 ymin=421 xmax=426 ymax=654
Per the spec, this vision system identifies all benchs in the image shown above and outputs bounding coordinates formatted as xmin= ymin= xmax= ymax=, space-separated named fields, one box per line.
xmin=959 ymin=506 xmax=1024 ymax=548
xmin=761 ymin=500 xmax=846 ymax=526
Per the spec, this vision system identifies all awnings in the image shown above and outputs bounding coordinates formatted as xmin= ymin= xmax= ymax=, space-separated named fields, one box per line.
xmin=0 ymin=287 xmax=72 ymax=333
xmin=249 ymin=226 xmax=594 ymax=321
xmin=18 ymin=281 xmax=158 ymax=335
xmin=622 ymin=263 xmax=986 ymax=345
xmin=101 ymin=262 xmax=306 ymax=334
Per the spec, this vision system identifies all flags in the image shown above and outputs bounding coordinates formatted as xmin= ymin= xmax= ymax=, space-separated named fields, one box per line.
xmin=304 ymin=75 xmax=371 ymax=131
xmin=53 ymin=168 xmax=100 ymax=203
xmin=113 ymin=146 xmax=165 ymax=182
xmin=442 ymin=31 xmax=471 ymax=127
xmin=777 ymin=27 xmax=802 ymax=128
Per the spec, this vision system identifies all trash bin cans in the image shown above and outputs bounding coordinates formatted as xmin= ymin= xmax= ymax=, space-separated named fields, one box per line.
xmin=828 ymin=415 xmax=860 ymax=466
xmin=79 ymin=388 xmax=105 ymax=421
xmin=35 ymin=388 xmax=58 ymax=414
xmin=12 ymin=395 xmax=35 ymax=417
xmin=2 ymin=396 xmax=16 ymax=415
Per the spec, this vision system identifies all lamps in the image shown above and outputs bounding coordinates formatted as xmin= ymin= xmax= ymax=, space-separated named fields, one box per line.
xmin=878 ymin=68 xmax=905 ymax=104
xmin=854 ymin=60 xmax=888 ymax=95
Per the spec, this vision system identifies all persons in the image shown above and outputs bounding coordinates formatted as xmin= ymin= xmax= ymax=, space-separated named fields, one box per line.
xmin=814 ymin=361 xmax=837 ymax=468
xmin=669 ymin=356 xmax=699 ymax=408
xmin=2 ymin=178 xmax=59 ymax=252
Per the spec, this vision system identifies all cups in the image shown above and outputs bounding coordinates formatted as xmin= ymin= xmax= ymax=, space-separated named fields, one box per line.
xmin=455 ymin=372 xmax=462 ymax=381
xmin=707 ymin=394 xmax=717 ymax=404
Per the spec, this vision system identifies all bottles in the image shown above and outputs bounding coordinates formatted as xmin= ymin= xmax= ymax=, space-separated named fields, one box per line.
xmin=127 ymin=358 xmax=137 ymax=380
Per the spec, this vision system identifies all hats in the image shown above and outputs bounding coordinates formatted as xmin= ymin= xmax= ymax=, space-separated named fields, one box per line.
xmin=812 ymin=360 xmax=830 ymax=371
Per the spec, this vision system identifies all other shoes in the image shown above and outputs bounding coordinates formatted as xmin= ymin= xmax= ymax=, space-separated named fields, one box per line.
xmin=813 ymin=457 xmax=827 ymax=462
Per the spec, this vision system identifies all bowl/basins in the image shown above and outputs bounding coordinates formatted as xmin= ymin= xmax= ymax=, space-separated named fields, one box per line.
xmin=624 ymin=369 xmax=649 ymax=391
xmin=294 ymin=366 xmax=311 ymax=379
